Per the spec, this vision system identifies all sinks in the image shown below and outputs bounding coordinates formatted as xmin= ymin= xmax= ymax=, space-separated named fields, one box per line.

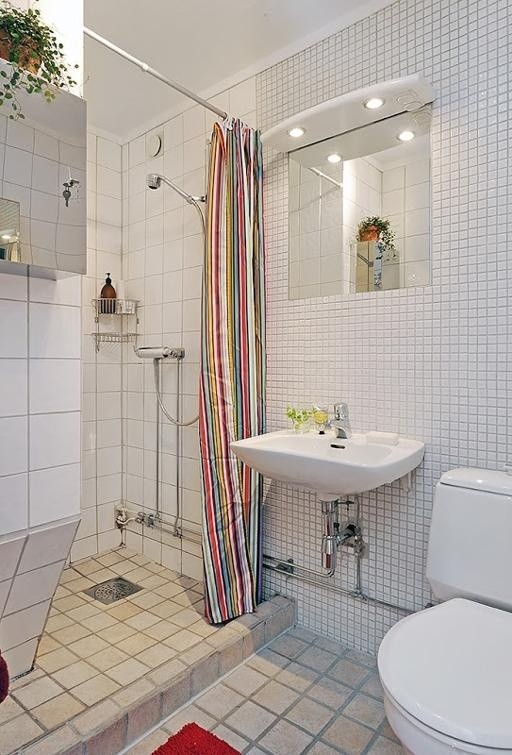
xmin=228 ymin=428 xmax=425 ymax=492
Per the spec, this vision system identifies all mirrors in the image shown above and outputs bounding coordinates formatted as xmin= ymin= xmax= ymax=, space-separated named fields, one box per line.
xmin=287 ymin=103 xmax=432 ymax=299
xmin=1 ymin=197 xmax=22 ymax=263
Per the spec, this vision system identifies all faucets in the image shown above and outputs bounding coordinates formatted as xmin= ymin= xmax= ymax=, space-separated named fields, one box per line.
xmin=327 ymin=402 xmax=353 ymax=440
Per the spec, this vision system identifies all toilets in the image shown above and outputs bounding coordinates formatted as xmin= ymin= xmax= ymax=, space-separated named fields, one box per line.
xmin=375 ymin=469 xmax=512 ymax=754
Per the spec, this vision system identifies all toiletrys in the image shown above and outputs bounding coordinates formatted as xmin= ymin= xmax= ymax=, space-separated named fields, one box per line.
xmin=116 ymin=278 xmax=126 ymax=314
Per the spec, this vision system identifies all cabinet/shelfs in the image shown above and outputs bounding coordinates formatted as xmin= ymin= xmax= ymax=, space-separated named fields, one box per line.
xmin=92 ymin=298 xmax=139 ymax=354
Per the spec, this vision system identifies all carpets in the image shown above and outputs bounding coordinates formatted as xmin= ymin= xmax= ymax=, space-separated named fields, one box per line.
xmin=148 ymin=723 xmax=244 ymax=755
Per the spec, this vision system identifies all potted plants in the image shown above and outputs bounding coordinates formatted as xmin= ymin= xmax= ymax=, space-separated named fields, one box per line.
xmin=356 ymin=216 xmax=397 ymax=263
xmin=0 ymin=0 xmax=79 ymax=122
